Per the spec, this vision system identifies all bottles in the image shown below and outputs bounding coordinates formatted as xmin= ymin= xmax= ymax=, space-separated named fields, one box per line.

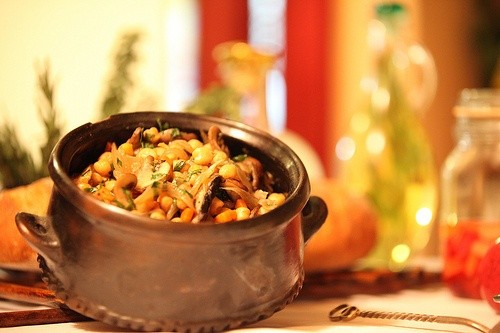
xmin=439 ymin=88 xmax=500 ymax=302
xmin=332 ymin=2 xmax=437 ymax=270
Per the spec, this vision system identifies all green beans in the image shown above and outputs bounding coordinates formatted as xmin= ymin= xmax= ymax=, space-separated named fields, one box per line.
xmin=76 ymin=127 xmax=287 ymax=221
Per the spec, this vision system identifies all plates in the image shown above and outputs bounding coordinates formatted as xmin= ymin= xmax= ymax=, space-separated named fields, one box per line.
xmin=0 ymin=262 xmax=43 ymax=284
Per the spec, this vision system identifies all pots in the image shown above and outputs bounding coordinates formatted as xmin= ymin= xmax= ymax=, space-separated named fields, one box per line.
xmin=14 ymin=111 xmax=329 ymax=333
